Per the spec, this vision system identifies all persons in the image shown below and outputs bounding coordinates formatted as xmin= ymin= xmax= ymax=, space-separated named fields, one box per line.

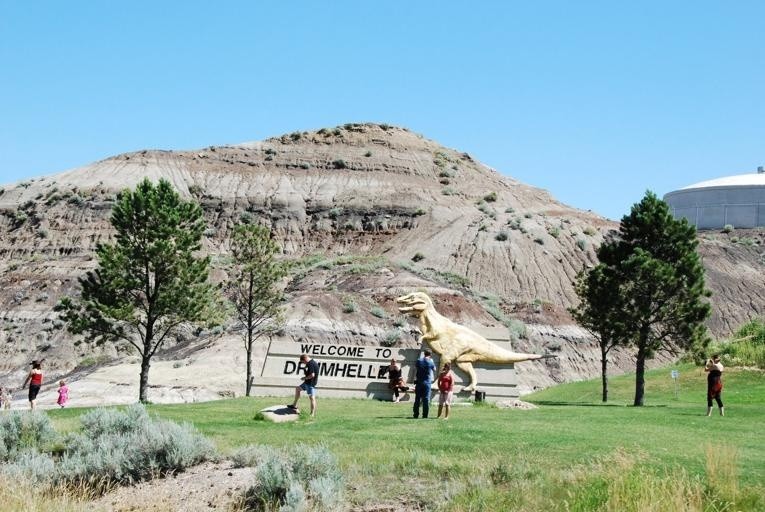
xmin=413 ymin=348 xmax=436 ymax=419
xmin=23 ymin=361 xmax=43 ymax=410
xmin=381 ymin=358 xmax=409 ymax=404
xmin=705 ymin=354 xmax=725 ymax=416
xmin=437 ymin=362 xmax=453 ymax=420
xmin=287 ymin=354 xmax=319 ymax=417
xmin=57 ymin=380 xmax=69 ymax=408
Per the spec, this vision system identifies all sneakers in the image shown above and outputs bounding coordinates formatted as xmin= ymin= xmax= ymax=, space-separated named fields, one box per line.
xmin=287 ymin=405 xmax=297 ymax=409
